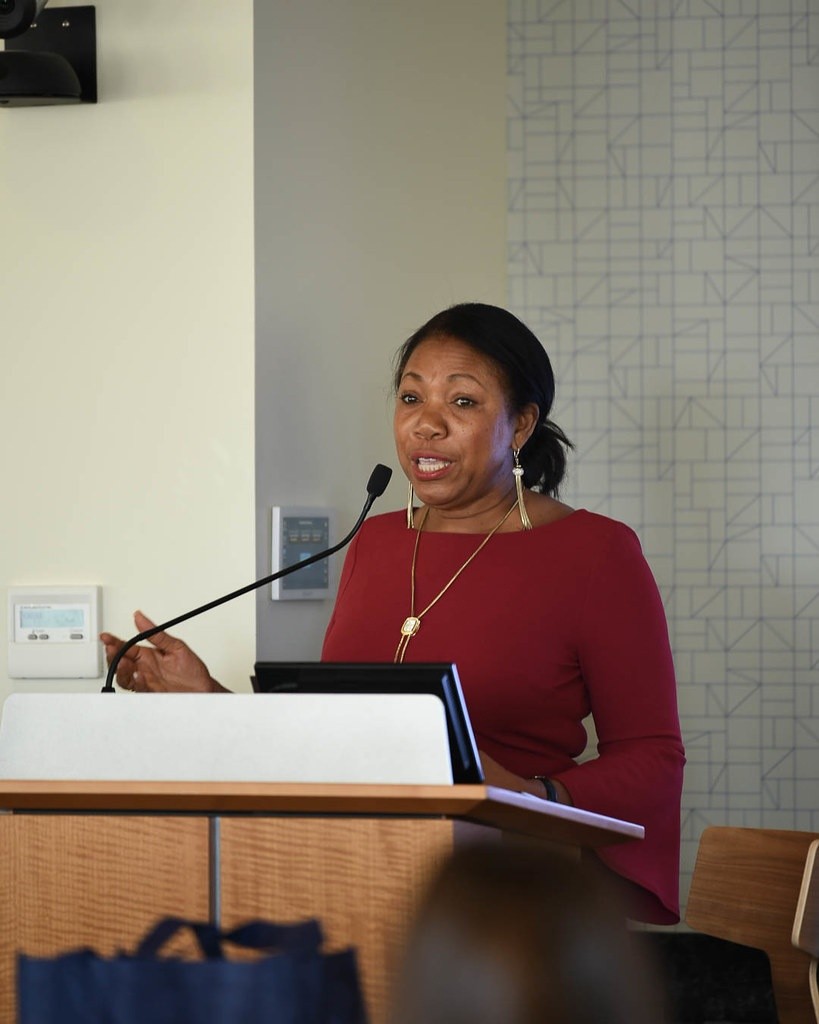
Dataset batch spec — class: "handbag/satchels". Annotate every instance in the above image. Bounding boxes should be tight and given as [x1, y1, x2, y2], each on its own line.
[17, 917, 369, 1024]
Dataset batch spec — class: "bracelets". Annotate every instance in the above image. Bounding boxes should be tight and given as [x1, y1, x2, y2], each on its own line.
[531, 775, 558, 803]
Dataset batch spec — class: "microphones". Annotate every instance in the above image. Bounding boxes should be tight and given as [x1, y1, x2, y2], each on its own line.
[101, 464, 392, 691]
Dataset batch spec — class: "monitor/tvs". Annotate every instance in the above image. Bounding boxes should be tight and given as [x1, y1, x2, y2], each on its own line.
[249, 661, 485, 785]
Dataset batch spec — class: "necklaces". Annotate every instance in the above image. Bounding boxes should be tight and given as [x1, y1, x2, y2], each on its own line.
[394, 486, 526, 664]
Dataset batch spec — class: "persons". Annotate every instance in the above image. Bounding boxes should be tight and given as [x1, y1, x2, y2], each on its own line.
[97, 303, 692, 927]
[384, 834, 684, 1024]
